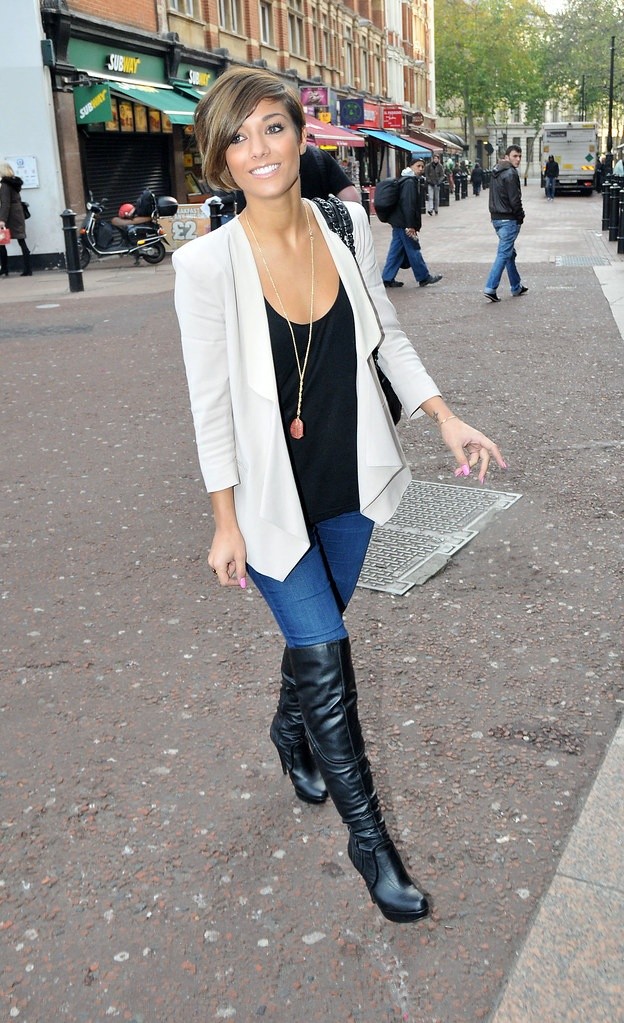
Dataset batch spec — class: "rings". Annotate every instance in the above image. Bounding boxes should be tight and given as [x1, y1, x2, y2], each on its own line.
[212, 568, 218, 574]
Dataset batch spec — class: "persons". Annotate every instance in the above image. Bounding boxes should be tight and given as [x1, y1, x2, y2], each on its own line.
[0, 162, 33, 278]
[543, 154, 559, 201]
[425, 154, 484, 216]
[167, 65, 506, 928]
[594, 154, 607, 193]
[484, 143, 530, 302]
[382, 157, 443, 288]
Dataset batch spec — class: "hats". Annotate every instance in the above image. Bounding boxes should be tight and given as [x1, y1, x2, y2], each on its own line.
[410, 158, 424, 165]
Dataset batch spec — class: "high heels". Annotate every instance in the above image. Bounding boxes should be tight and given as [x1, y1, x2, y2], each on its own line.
[20, 268, 32, 277]
[0, 266, 8, 276]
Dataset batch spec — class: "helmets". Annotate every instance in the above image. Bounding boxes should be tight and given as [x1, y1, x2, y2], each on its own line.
[119, 204, 136, 218]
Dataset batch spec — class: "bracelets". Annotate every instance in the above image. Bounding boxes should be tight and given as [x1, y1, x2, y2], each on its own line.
[440, 415, 457, 425]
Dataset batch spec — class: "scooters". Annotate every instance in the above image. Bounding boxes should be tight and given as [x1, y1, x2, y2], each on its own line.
[79, 188, 180, 266]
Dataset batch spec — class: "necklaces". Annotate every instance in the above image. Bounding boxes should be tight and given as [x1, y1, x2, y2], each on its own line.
[245, 196, 315, 440]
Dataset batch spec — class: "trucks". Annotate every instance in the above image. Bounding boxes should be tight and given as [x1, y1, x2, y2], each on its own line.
[540, 123, 599, 196]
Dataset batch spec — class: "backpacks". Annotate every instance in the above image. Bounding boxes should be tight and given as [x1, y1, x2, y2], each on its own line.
[373, 176, 415, 222]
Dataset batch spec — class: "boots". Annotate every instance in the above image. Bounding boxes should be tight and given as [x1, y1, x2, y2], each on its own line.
[269, 640, 329, 804]
[290, 636, 428, 922]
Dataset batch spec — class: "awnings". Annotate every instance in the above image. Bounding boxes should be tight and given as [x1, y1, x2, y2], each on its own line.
[95, 79, 464, 160]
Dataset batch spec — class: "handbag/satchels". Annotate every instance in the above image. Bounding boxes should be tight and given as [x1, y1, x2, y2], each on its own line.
[21, 201, 30, 218]
[0, 226, 10, 244]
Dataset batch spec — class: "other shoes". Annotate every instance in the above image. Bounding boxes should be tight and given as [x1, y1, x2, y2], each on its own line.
[520, 286, 528, 293]
[428, 211, 432, 215]
[485, 292, 502, 302]
[383, 279, 404, 288]
[435, 211, 438, 214]
[420, 274, 443, 287]
